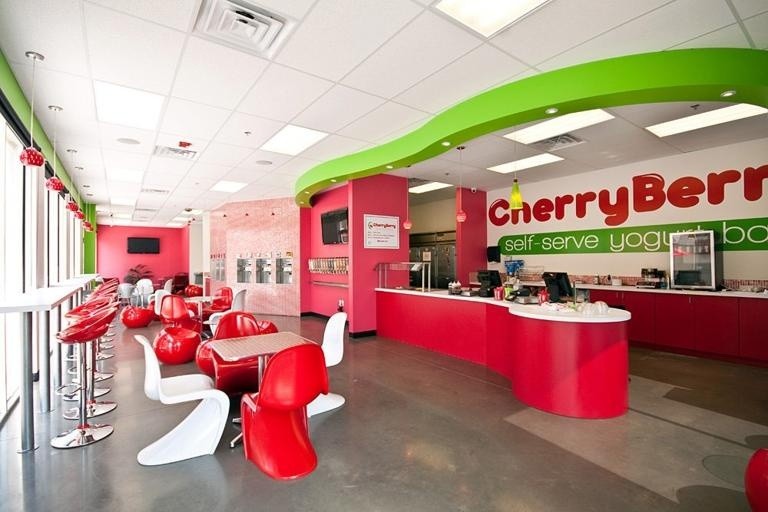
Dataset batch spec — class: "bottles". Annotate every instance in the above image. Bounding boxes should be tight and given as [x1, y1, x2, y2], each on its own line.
[503, 278, 521, 299]
[593, 274, 599, 285]
[448, 280, 462, 296]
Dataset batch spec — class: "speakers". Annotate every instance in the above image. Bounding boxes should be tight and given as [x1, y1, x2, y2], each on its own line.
[487, 247, 500, 262]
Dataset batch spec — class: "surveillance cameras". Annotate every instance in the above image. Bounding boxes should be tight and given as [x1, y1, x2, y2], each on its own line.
[470, 188, 477, 193]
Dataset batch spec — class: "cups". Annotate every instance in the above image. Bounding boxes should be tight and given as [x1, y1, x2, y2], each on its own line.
[582, 301, 609, 315]
[537, 293, 548, 306]
[494, 288, 504, 300]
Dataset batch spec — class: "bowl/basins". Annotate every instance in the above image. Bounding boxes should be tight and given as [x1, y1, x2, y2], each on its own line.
[612, 279, 622, 286]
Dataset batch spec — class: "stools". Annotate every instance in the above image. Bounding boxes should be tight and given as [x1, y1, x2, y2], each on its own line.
[49, 275, 117, 448]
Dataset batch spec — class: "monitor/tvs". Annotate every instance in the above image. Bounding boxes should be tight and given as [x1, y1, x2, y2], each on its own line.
[127, 237, 160, 254]
[542, 272, 573, 303]
[321, 208, 348, 244]
[477, 271, 502, 297]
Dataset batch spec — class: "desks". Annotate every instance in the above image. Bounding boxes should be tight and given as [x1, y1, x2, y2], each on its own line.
[2, 275, 98, 454]
[208, 331, 320, 450]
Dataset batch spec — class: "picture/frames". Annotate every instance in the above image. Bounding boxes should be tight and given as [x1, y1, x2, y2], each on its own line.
[363, 213, 400, 249]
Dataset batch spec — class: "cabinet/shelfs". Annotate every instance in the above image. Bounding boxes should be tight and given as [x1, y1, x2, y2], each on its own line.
[589, 289, 655, 349]
[655, 292, 738, 363]
[738, 298, 767, 370]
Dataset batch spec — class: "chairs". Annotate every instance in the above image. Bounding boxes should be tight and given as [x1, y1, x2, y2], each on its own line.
[238, 343, 330, 480]
[305, 310, 350, 418]
[132, 332, 231, 468]
[210, 311, 264, 390]
[118, 272, 279, 375]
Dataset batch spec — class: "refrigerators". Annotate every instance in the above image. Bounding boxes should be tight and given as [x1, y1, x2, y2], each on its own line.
[669, 230, 721, 291]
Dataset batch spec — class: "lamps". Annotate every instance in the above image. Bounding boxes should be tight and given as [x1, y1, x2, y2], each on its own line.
[403, 167, 412, 230]
[455, 146, 467, 223]
[20, 50, 94, 233]
[508, 124, 524, 211]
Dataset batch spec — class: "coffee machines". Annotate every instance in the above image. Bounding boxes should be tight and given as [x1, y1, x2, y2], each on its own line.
[636, 267, 660, 289]
